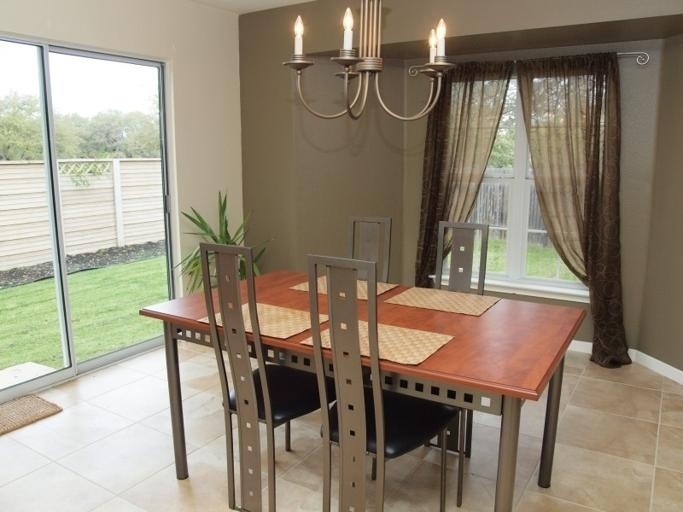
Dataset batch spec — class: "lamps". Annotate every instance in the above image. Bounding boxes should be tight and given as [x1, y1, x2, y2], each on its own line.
[283, 1, 457, 121]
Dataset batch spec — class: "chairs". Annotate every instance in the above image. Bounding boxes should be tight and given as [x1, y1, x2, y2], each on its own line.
[424, 220, 489, 459]
[347, 215, 393, 283]
[308, 255, 466, 512]
[199, 242, 337, 512]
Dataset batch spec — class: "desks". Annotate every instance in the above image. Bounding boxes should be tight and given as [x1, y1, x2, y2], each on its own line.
[139, 271, 587, 512]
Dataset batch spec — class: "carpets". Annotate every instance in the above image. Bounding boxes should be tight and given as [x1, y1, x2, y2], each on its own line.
[0, 393, 63, 437]
